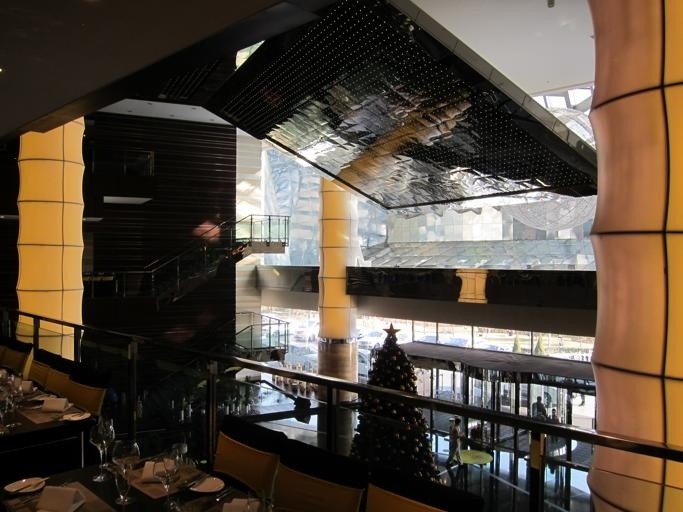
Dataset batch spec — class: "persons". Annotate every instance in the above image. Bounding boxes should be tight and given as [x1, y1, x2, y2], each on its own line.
[442, 414, 465, 466]
[548, 408, 562, 444]
[531, 394, 546, 421]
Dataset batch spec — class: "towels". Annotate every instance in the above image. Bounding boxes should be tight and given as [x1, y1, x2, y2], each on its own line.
[22, 380, 38, 393]
[36, 485, 86, 511]
[42, 398, 74, 412]
[138, 458, 176, 482]
[221, 495, 261, 511]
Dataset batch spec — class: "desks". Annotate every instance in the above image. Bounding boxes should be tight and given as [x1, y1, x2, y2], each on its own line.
[0, 451, 272, 511]
[0, 370, 92, 469]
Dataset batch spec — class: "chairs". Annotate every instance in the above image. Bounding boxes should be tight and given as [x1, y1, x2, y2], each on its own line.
[365, 483, 450, 511]
[269, 465, 366, 511]
[21, 358, 50, 386]
[0, 345, 24, 371]
[212, 431, 281, 499]
[43, 367, 69, 395]
[65, 381, 107, 421]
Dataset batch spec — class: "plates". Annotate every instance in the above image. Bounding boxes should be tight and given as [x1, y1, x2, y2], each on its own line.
[187, 474, 225, 494]
[4, 477, 47, 496]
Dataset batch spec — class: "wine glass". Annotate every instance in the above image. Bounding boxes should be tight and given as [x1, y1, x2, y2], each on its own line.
[151, 451, 183, 511]
[87, 414, 141, 505]
[0, 370, 23, 434]
[242, 498, 274, 512]
